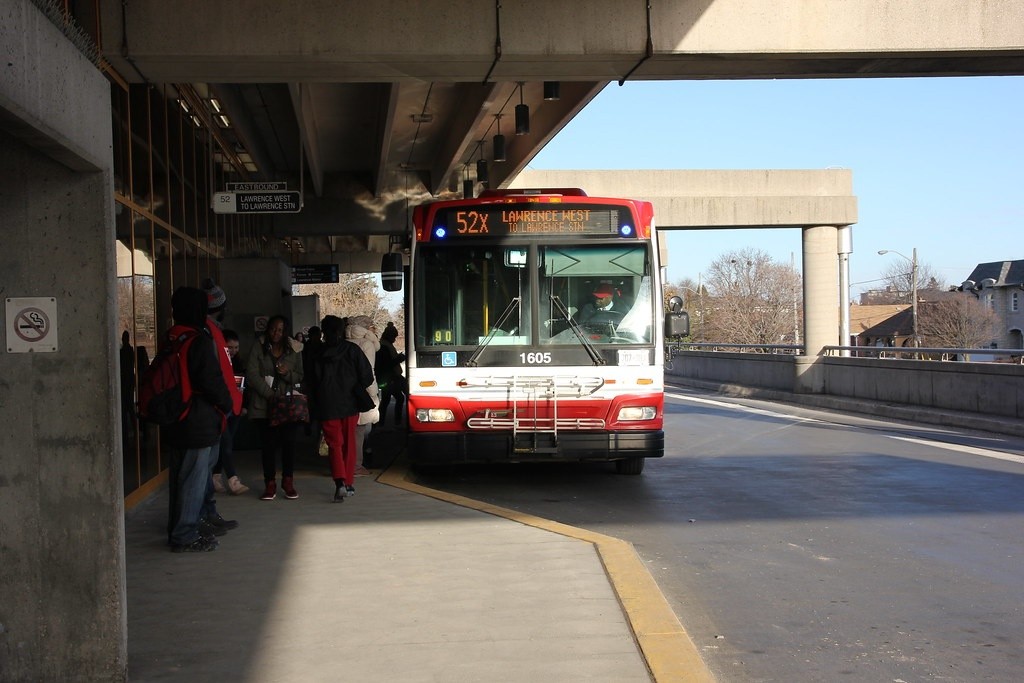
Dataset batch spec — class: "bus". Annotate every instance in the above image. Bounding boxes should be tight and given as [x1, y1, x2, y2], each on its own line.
[379, 186, 692, 477]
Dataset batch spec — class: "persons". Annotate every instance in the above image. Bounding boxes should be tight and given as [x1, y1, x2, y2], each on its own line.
[578, 282, 631, 325]
[295, 315, 374, 503]
[120, 330, 149, 449]
[375, 322, 408, 428]
[243, 315, 304, 500]
[158, 278, 250, 553]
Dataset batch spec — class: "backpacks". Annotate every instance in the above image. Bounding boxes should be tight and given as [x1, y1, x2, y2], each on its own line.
[137, 329, 201, 428]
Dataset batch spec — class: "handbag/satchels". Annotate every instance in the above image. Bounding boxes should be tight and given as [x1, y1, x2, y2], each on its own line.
[267, 369, 310, 428]
[312, 430, 329, 457]
[351, 382, 376, 412]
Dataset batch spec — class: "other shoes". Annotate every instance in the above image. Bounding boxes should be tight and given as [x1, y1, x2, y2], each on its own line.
[393, 423, 405, 432]
[173, 536, 216, 552]
[167, 531, 215, 546]
[334, 484, 346, 503]
[346, 486, 355, 495]
[378, 425, 390, 432]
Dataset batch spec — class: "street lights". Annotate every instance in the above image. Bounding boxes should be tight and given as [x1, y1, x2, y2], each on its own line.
[878, 247, 919, 361]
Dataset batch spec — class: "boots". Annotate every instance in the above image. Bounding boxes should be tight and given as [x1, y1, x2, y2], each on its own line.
[212, 473, 226, 495]
[198, 513, 226, 536]
[206, 500, 239, 531]
[227, 475, 248, 496]
[281, 476, 299, 500]
[261, 479, 276, 500]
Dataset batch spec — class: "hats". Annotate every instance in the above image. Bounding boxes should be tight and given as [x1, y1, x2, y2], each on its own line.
[592, 284, 615, 299]
[385, 322, 399, 336]
[202, 278, 227, 314]
